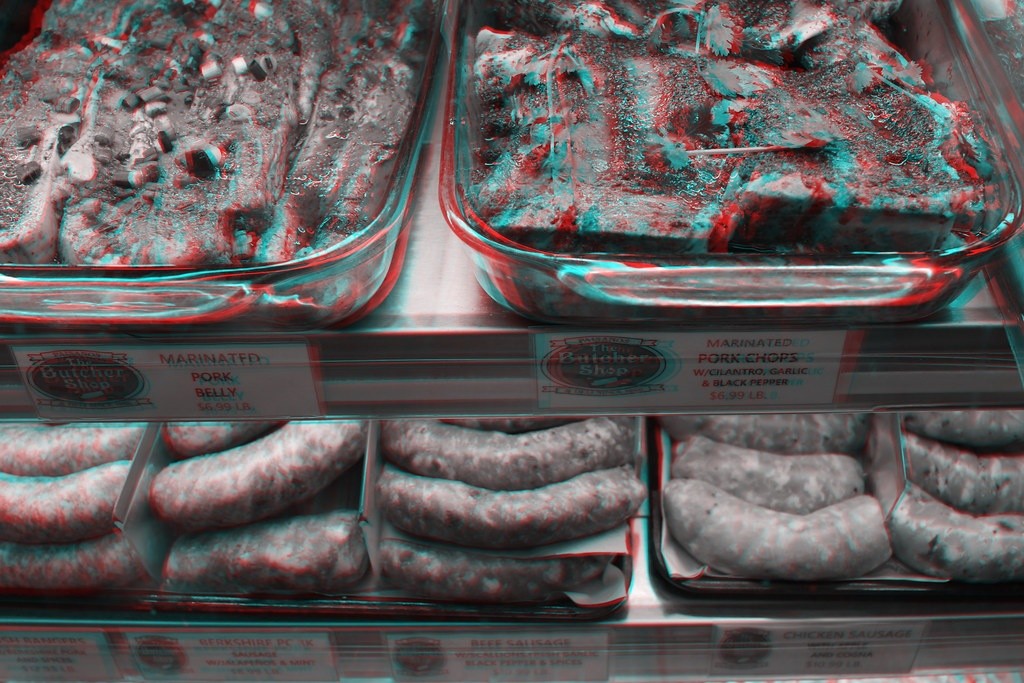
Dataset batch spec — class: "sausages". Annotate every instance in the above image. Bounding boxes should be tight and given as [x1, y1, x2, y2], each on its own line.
[0, 407, 1024, 603]
